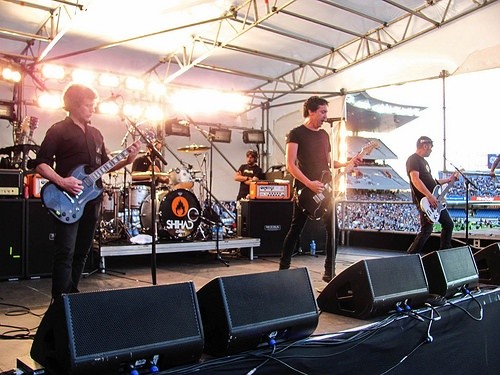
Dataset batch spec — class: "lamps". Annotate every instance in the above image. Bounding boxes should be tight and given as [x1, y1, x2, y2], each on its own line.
[243, 130, 265, 143]
[165, 119, 190, 136]
[210, 129, 232, 143]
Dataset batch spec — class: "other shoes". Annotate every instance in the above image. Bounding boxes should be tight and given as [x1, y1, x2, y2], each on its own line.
[322, 275, 337, 283]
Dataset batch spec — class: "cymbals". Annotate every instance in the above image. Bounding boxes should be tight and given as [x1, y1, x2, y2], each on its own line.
[177, 145, 211, 151]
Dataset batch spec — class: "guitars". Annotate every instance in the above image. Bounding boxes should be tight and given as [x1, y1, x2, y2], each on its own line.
[295, 139, 381, 221]
[40, 129, 157, 225]
[419, 168, 465, 224]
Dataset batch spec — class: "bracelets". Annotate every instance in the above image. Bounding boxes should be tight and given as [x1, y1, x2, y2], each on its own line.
[247, 176, 249, 180]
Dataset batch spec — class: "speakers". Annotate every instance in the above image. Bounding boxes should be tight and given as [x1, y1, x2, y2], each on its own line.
[196, 266, 320, 358]
[30, 280, 205, 375]
[294, 198, 329, 254]
[473, 240, 500, 286]
[238, 199, 295, 258]
[314, 252, 430, 321]
[422, 245, 480, 299]
[0, 199, 59, 279]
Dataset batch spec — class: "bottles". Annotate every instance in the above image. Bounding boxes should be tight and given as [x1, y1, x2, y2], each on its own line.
[212, 225, 217, 240]
[127, 226, 139, 243]
[217, 223, 223, 241]
[310, 240, 316, 256]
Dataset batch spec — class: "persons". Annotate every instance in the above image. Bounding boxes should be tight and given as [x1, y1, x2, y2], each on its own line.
[279, 96, 339, 283]
[132, 144, 162, 172]
[337, 166, 500, 241]
[33, 86, 141, 297]
[406, 137, 455, 255]
[235, 150, 262, 200]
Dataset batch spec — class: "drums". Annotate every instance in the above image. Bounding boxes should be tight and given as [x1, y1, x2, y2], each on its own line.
[167, 167, 194, 190]
[139, 188, 201, 241]
[121, 184, 159, 210]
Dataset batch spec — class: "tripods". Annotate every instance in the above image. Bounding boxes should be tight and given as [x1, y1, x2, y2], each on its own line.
[81, 187, 126, 279]
[198, 215, 231, 266]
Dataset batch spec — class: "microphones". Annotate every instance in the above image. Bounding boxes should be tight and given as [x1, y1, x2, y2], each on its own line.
[323, 117, 345, 122]
[96, 94, 119, 107]
[190, 211, 199, 217]
